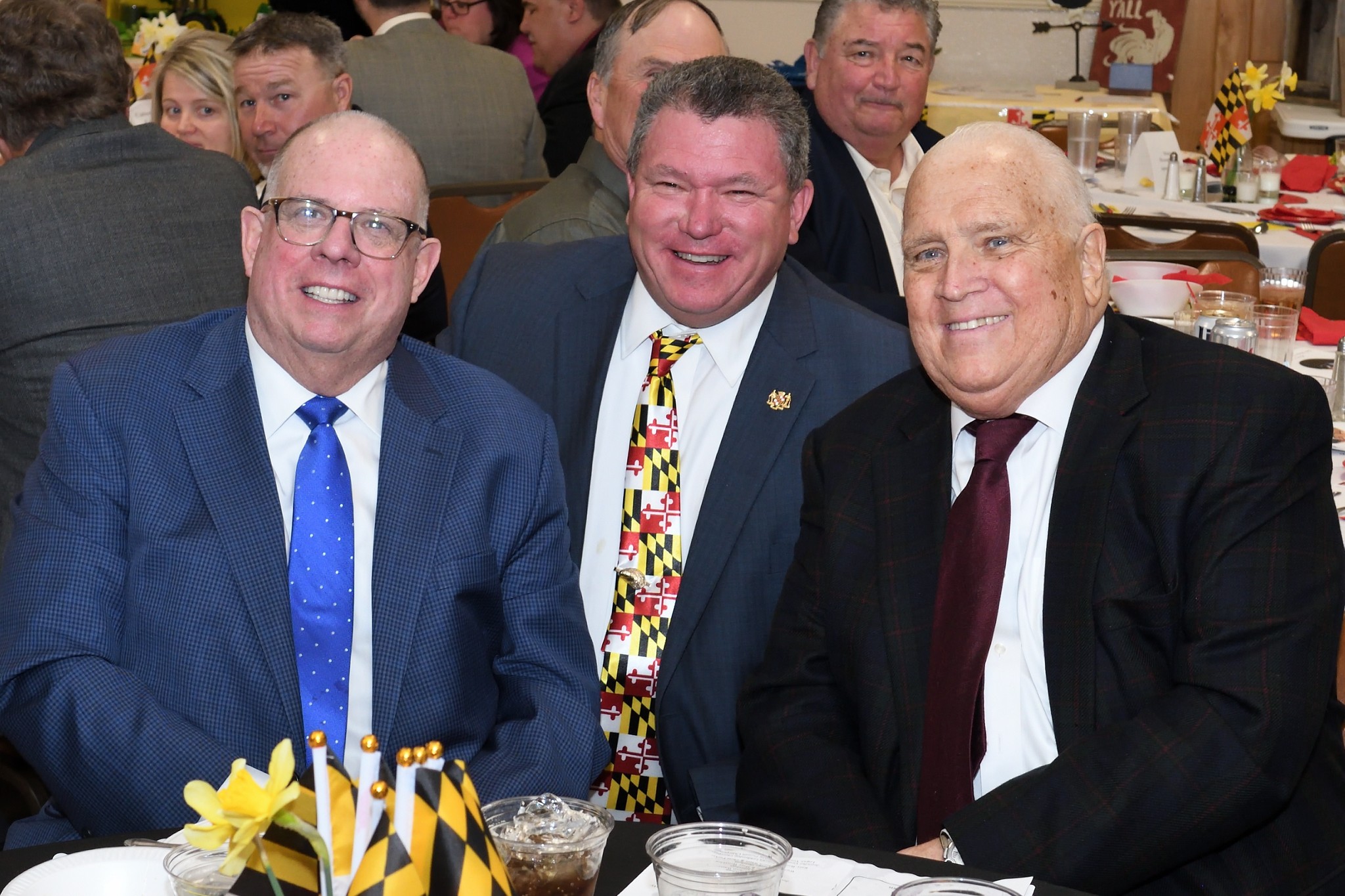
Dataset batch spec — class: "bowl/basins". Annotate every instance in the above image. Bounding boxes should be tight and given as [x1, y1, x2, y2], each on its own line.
[1105, 261, 1204, 319]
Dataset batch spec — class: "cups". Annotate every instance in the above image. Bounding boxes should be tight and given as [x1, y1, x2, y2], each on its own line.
[1245, 304, 1300, 368]
[1067, 112, 1103, 180]
[1310, 375, 1337, 414]
[1258, 165, 1282, 199]
[481, 795, 615, 896]
[1258, 267, 1309, 315]
[890, 877, 1023, 896]
[1114, 134, 1141, 177]
[1189, 289, 1256, 321]
[1335, 138, 1345, 178]
[645, 822, 793, 896]
[1236, 172, 1260, 204]
[163, 835, 265, 896]
[1173, 309, 1201, 336]
[1252, 145, 1288, 174]
[1118, 111, 1152, 144]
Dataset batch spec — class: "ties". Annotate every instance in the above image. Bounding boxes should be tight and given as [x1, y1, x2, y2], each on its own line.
[914, 412, 1040, 845]
[579, 330, 705, 824]
[284, 396, 357, 765]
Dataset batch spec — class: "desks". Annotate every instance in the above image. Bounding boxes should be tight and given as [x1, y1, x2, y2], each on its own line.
[1065, 149, 1345, 269]
[921, 79, 1176, 163]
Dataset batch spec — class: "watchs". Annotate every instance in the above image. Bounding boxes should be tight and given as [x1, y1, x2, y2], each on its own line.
[939, 833, 965, 866]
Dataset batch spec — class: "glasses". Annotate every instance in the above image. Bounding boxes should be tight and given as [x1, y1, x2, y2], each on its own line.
[259, 192, 429, 261]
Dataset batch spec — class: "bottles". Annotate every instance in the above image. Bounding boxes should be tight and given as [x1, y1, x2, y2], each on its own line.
[1191, 157, 1208, 202]
[1161, 151, 1182, 201]
[1328, 335, 1345, 421]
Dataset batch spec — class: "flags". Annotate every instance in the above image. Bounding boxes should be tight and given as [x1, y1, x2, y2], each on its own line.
[229, 732, 511, 896]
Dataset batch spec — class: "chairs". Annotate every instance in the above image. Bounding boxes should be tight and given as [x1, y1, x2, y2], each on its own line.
[1103, 246, 1268, 300]
[424, 172, 554, 305]
[1089, 211, 1262, 260]
[1303, 228, 1345, 319]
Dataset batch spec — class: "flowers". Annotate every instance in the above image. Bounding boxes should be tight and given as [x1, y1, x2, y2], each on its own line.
[133, 9, 188, 57]
[1237, 59, 1300, 119]
[167, 736, 334, 896]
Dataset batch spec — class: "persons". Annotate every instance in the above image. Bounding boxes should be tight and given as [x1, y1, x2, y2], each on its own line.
[432, 56, 916, 826]
[225, 14, 448, 345]
[486, 0, 730, 246]
[338, 0, 621, 208]
[150, 30, 257, 182]
[775, 0, 945, 315]
[0, 112, 610, 846]
[737, 122, 1344, 896]
[0, 0, 259, 548]
